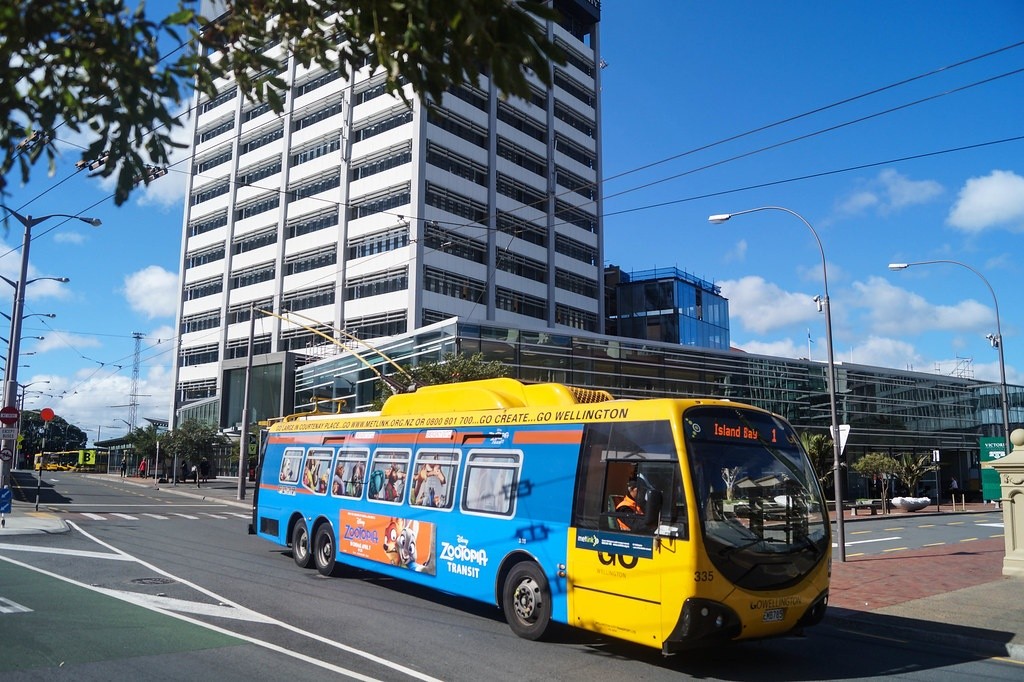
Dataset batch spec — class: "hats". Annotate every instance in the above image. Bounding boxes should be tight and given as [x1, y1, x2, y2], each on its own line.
[628, 477, 651, 491]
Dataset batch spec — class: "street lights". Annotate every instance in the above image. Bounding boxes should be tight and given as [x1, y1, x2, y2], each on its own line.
[887, 260, 1011, 458]
[0, 203, 103, 489]
[707, 205, 846, 563]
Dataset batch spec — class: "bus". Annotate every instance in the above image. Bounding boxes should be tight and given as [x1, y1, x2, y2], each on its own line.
[246, 299, 833, 660]
[33, 448, 109, 473]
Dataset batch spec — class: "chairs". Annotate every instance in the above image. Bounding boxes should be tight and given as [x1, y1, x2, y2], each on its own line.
[607, 494, 629, 531]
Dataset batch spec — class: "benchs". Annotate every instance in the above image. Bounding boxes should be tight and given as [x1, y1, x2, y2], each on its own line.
[848, 504, 881, 516]
[856, 499, 892, 509]
[811, 500, 848, 511]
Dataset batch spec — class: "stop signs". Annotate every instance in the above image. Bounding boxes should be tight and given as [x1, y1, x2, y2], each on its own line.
[0, 406, 20, 425]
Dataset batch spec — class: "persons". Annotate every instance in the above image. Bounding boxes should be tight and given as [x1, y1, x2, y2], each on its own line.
[616, 474, 662, 532]
[180, 460, 187, 483]
[948, 476, 958, 503]
[120, 459, 126, 478]
[200, 457, 210, 483]
[192, 464, 197, 483]
[139, 458, 146, 479]
